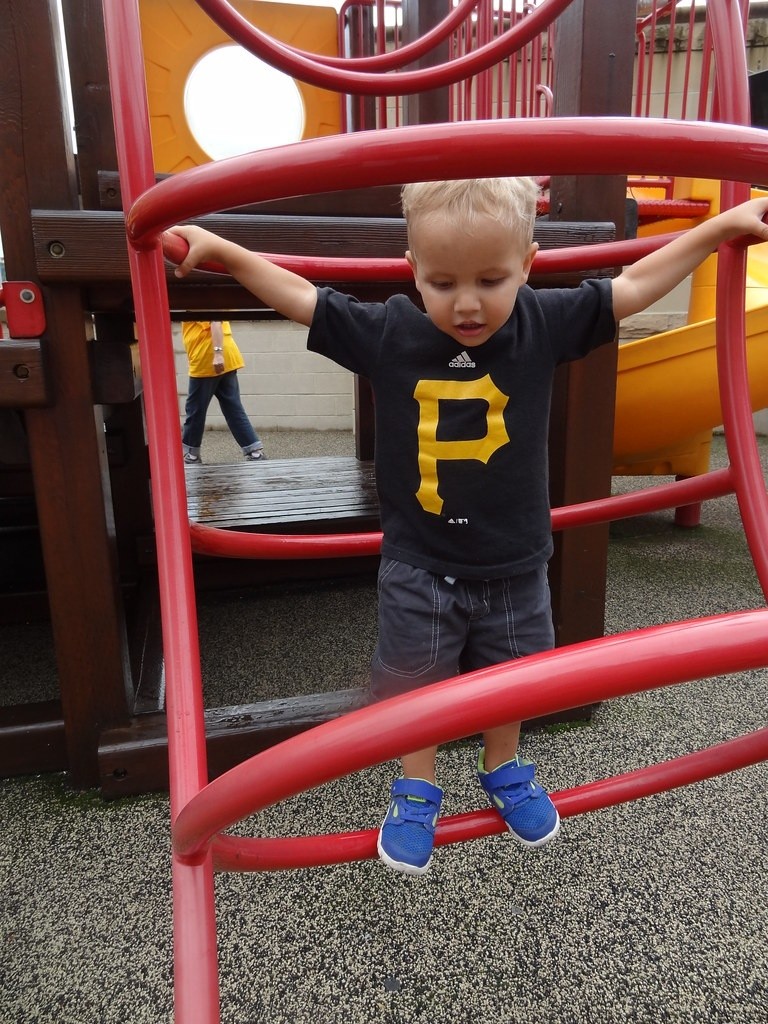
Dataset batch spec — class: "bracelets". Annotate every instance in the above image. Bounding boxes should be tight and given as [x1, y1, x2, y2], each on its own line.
[214, 347, 222, 351]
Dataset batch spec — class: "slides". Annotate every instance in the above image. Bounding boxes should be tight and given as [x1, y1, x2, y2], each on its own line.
[614, 176, 768, 478]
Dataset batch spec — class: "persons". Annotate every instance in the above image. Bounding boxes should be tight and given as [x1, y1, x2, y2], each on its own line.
[182, 321, 267, 464]
[167, 177, 768, 875]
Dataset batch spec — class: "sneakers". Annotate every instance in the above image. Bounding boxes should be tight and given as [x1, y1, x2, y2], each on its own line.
[376, 772, 445, 877]
[476, 744, 560, 847]
[183, 452, 203, 465]
[246, 451, 268, 461]
